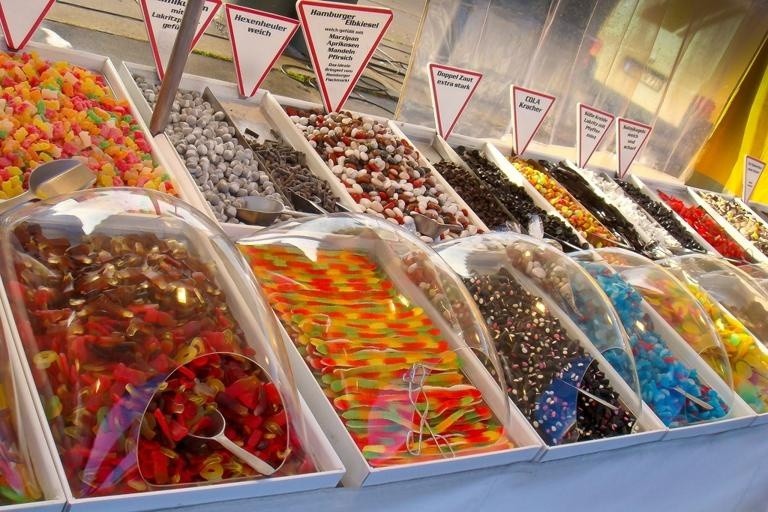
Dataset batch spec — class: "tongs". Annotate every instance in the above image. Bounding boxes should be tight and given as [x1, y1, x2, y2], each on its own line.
[400, 364, 453, 460]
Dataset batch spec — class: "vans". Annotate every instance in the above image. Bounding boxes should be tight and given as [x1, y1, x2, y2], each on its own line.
[578, 0, 752, 146]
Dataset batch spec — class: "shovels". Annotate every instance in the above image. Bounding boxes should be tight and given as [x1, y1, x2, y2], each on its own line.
[414, 214, 461, 239]
[186, 408, 276, 476]
[234, 196, 318, 226]
[0, 160, 97, 216]
[288, 188, 353, 214]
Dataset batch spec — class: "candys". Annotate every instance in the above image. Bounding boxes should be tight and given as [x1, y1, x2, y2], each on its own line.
[0, 382, 43, 507]
[553, 259, 734, 429]
[403, 253, 642, 448]
[655, 190, 750, 258]
[459, 144, 590, 248]
[0, 220, 316, 497]
[630, 276, 768, 415]
[430, 154, 512, 233]
[283, 106, 484, 245]
[0, 47, 181, 202]
[510, 156, 624, 248]
[238, 244, 518, 470]
[616, 179, 707, 250]
[132, 72, 296, 225]
[704, 192, 768, 257]
[538, 159, 645, 249]
[580, 168, 681, 248]
